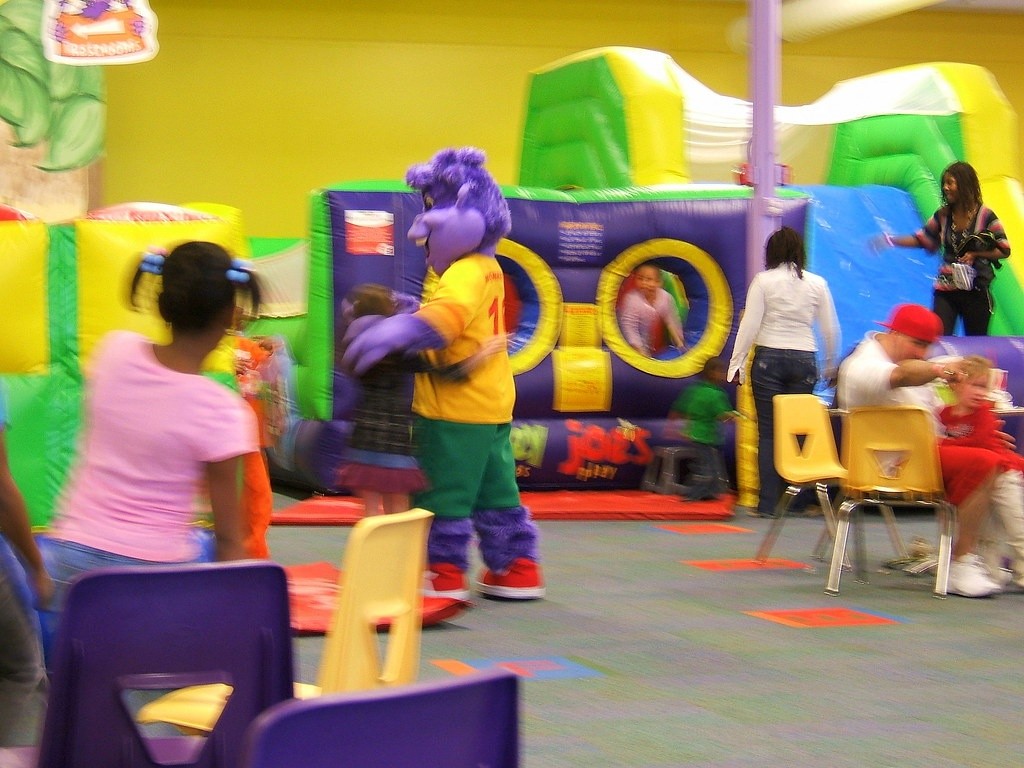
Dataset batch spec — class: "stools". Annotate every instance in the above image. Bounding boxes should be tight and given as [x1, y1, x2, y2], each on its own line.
[644, 445, 728, 496]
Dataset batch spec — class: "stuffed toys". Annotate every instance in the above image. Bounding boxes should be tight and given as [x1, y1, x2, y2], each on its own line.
[340, 146, 544, 600]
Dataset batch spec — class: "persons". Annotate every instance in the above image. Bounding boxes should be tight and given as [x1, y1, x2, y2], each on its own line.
[871, 162, 1011, 336]
[40, 241, 261, 609]
[838, 303, 1016, 598]
[621, 265, 685, 358]
[237, 333, 272, 559]
[0, 382, 55, 747]
[663, 357, 737, 499]
[936, 348, 1024, 587]
[343, 285, 515, 518]
[727, 227, 842, 519]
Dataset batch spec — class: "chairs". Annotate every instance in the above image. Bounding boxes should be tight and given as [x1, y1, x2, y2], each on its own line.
[1, 559, 294, 768]
[237, 672, 518, 768]
[749, 394, 911, 573]
[824, 407, 957, 601]
[134, 508, 434, 739]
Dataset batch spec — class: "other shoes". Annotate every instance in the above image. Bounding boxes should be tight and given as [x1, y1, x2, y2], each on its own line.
[744, 506, 778, 519]
[951, 263, 975, 291]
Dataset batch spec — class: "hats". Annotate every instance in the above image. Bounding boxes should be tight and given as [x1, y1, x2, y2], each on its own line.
[873, 305, 939, 344]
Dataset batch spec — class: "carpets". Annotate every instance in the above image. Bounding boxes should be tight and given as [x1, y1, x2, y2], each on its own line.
[266, 494, 735, 526]
[275, 559, 474, 635]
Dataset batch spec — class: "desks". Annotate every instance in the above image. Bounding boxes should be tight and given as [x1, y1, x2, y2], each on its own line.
[989, 404, 1024, 418]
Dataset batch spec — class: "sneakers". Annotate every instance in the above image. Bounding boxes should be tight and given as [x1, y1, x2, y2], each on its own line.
[947, 553, 1001, 597]
